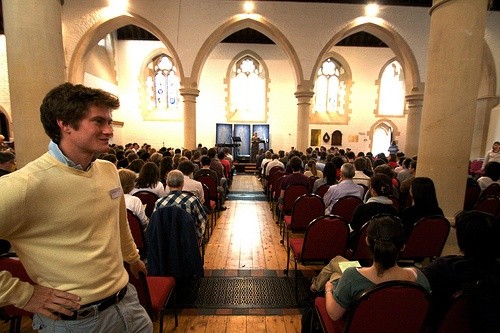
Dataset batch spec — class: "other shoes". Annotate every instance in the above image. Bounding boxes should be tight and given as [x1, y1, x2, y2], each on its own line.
[220, 206, 227, 210]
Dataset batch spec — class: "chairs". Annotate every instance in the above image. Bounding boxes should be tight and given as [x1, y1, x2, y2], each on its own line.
[260, 162, 500, 333]
[126, 162, 229, 333]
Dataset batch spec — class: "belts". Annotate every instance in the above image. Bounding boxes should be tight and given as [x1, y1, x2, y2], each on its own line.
[54, 286, 129, 320]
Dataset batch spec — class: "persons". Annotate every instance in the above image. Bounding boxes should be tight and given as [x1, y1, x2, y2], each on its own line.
[117, 169, 150, 229]
[400, 176, 443, 253]
[463, 140, 500, 211]
[256, 146, 417, 202]
[389, 141, 400, 154]
[349, 174, 403, 253]
[0, 134, 16, 254]
[251, 133, 261, 163]
[99, 143, 234, 211]
[310, 214, 431, 322]
[151, 168, 208, 247]
[0, 83, 154, 333]
[324, 163, 365, 217]
[432, 210, 500, 333]
